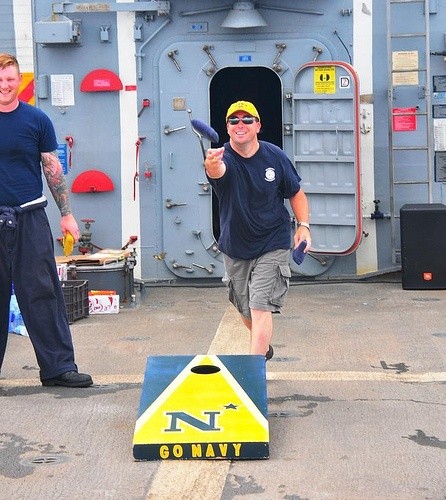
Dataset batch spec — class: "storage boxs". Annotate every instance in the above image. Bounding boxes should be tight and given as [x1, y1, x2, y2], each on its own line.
[60, 279, 89, 324]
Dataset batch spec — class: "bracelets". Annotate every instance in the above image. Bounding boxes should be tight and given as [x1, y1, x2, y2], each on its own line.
[297, 222, 310, 232]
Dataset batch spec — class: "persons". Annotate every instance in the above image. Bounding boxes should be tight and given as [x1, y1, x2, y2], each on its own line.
[205, 100, 312, 359]
[0, 52, 94, 389]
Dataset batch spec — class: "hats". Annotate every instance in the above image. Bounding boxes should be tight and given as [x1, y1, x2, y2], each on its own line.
[225, 100, 260, 123]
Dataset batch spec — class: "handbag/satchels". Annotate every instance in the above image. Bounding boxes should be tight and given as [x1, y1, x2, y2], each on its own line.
[7, 293, 27, 335]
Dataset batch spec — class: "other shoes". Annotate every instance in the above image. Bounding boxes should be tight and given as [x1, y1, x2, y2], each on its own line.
[42, 370, 93, 386]
[265, 345, 273, 359]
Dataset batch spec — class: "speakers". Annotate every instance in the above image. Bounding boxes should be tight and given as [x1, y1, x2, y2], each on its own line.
[399, 203, 446, 290]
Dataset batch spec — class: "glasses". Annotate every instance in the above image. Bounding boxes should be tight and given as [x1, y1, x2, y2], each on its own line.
[226, 115, 255, 125]
